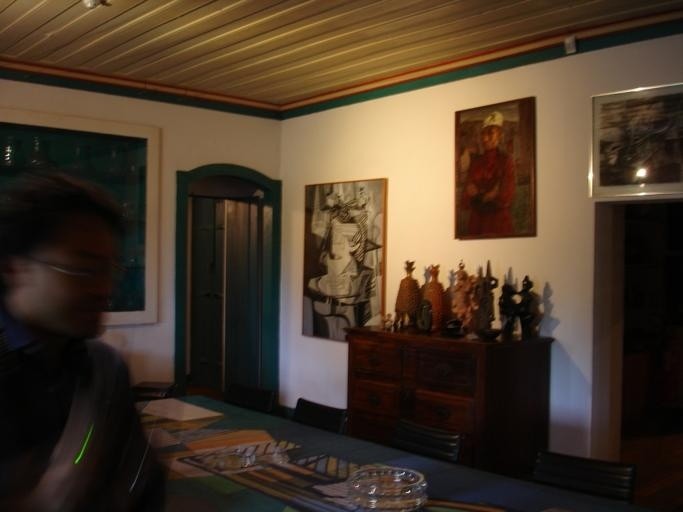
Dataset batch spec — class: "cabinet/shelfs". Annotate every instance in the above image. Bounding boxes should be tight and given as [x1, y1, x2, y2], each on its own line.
[342, 321, 554, 458]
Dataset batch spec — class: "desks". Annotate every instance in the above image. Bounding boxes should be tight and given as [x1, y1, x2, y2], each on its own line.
[134, 390, 636, 512]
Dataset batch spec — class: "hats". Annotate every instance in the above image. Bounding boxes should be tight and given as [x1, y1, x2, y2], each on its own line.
[483, 111, 504, 128]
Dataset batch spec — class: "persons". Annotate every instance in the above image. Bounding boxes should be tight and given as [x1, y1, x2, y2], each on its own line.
[2, 165, 165, 510]
[460, 112, 516, 236]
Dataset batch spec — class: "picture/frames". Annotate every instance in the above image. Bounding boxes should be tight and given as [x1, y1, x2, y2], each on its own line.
[301, 178, 388, 344]
[0, 108, 161, 327]
[588, 82, 683, 198]
[453, 95, 536, 237]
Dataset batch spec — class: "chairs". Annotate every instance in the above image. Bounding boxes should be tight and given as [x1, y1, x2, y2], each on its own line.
[531, 448, 636, 505]
[293, 397, 347, 436]
[395, 418, 464, 466]
[222, 382, 272, 413]
